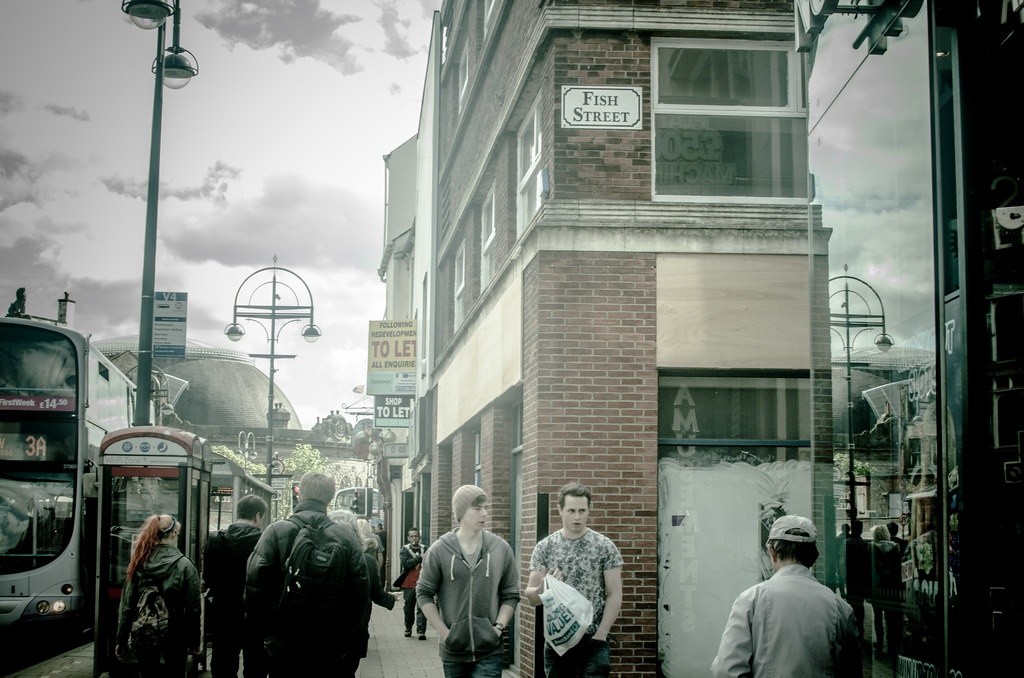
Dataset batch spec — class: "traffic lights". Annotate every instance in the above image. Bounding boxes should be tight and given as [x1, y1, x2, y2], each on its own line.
[350, 487, 367, 517]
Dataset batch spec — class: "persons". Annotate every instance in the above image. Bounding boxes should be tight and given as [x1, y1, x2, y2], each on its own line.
[525, 482, 623, 678]
[710, 515, 859, 678]
[330, 504, 402, 623]
[836, 523, 913, 668]
[414, 484, 521, 678]
[115, 514, 201, 678]
[245, 470, 369, 678]
[201, 495, 268, 678]
[393, 527, 429, 640]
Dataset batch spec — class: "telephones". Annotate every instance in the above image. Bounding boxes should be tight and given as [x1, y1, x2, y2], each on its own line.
[110, 527, 140, 584]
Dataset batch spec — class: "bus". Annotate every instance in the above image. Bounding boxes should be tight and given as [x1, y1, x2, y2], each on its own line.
[0, 288, 155, 624]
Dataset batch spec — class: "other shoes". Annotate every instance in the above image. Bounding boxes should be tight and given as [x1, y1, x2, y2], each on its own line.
[404, 626, 412, 636]
[418, 633, 426, 640]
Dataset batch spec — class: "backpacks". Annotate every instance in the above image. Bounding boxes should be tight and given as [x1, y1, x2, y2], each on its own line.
[128, 553, 186, 654]
[263, 517, 346, 642]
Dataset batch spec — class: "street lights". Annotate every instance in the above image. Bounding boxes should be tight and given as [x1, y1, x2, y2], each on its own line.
[827, 264, 894, 531]
[224, 255, 322, 486]
[122, 0, 200, 430]
[235, 431, 257, 461]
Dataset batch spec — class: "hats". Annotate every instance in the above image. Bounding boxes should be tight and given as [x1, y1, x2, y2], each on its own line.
[766, 515, 816, 543]
[452, 485, 487, 522]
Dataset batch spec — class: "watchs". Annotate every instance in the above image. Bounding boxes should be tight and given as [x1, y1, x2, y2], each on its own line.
[492, 623, 504, 632]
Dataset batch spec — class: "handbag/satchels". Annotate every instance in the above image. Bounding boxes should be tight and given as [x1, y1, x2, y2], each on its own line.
[539, 573, 594, 657]
